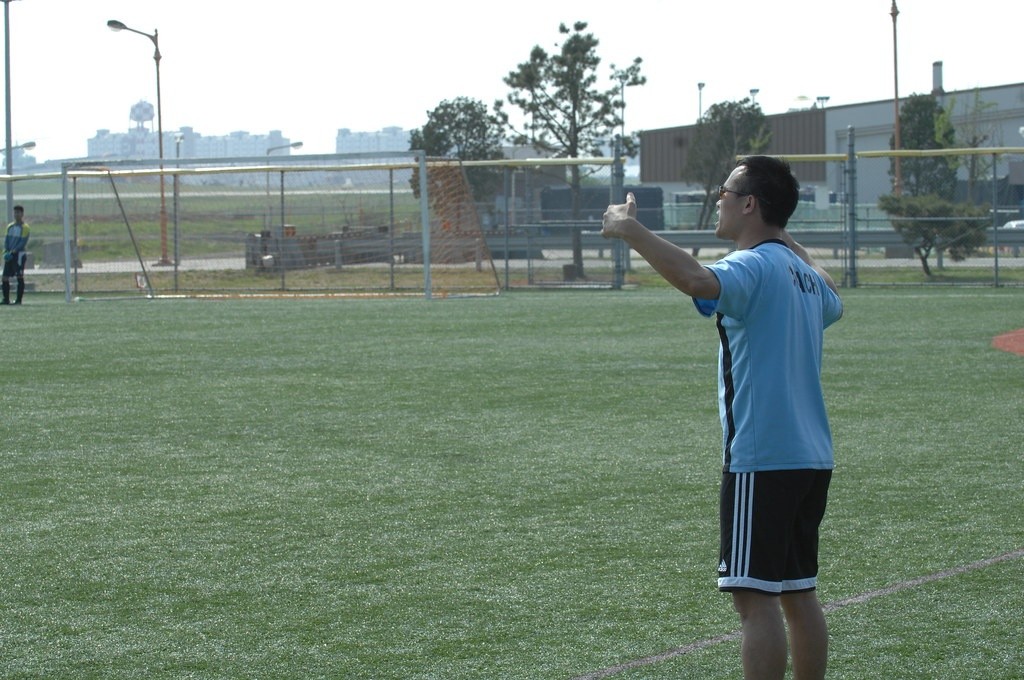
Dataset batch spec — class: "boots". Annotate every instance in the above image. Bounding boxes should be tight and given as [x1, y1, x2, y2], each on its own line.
[10, 283, 25, 305]
[0, 283, 9, 305]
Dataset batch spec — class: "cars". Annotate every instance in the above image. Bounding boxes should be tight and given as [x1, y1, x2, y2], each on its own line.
[1003, 220, 1024, 228]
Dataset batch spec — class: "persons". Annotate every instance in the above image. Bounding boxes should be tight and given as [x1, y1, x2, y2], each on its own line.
[0, 205, 30, 306]
[600, 155, 843, 680]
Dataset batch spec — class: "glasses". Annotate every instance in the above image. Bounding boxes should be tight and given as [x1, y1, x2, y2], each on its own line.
[718, 185, 750, 199]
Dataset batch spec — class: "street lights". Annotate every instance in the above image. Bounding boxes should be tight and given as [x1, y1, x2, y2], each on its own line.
[620, 75, 625, 146]
[106, 19, 168, 265]
[698, 84, 705, 124]
[263, 140, 301, 230]
[818, 97, 830, 107]
[175, 135, 183, 224]
[751, 88, 760, 110]
